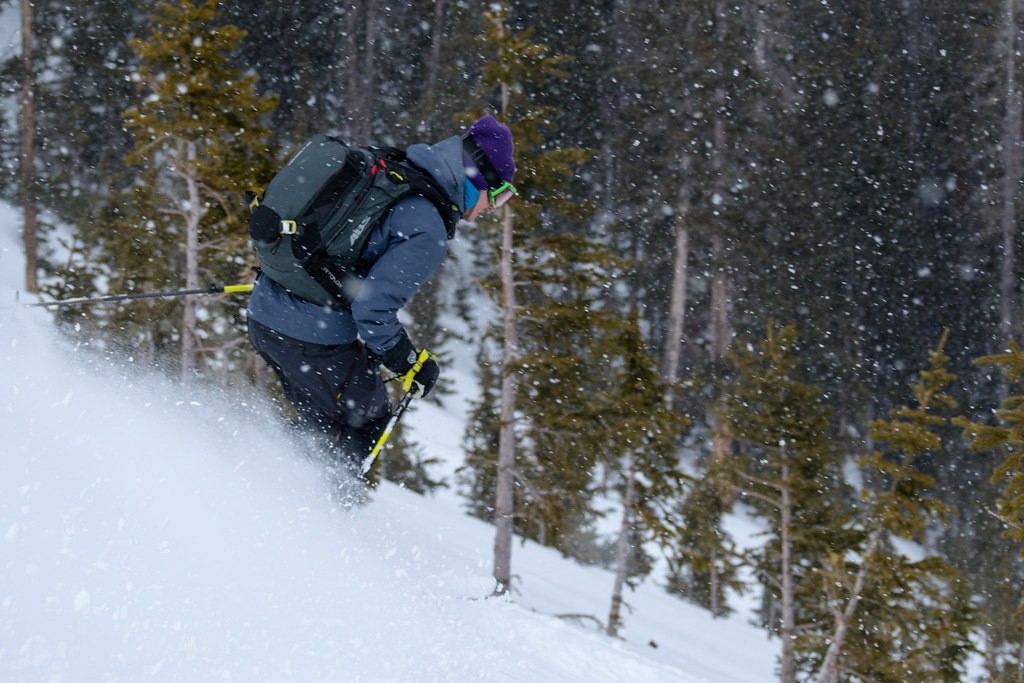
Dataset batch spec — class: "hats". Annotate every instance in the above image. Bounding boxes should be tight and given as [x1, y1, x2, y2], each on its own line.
[464, 115, 516, 192]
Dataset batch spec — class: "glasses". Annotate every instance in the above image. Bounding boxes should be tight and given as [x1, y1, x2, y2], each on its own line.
[488, 182, 515, 210]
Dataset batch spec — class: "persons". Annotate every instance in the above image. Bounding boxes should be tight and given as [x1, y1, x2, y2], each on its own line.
[245, 111, 516, 514]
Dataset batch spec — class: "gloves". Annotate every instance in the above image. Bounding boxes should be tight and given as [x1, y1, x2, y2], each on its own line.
[365, 327, 439, 400]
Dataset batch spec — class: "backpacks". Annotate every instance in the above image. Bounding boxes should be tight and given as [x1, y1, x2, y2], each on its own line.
[247, 132, 457, 311]
